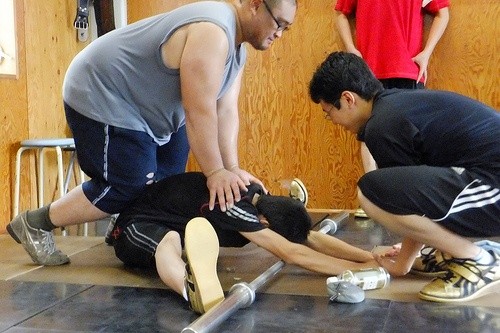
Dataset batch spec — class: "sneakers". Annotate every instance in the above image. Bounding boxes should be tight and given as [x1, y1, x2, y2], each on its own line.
[289, 178, 308, 209]
[410, 247, 454, 275]
[6, 209, 70, 266]
[417, 250, 500, 303]
[184, 217, 224, 314]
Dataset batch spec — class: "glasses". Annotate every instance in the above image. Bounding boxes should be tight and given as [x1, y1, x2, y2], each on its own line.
[325, 100, 339, 120]
[263, 0, 288, 30]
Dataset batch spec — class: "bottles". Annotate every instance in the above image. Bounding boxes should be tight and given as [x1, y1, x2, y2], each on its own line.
[341, 267, 391, 291]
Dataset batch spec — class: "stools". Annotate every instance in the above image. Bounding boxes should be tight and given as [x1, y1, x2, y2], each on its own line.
[13, 137, 88, 236]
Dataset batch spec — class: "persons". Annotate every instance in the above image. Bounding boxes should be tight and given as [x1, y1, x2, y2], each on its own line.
[6, 0, 298, 267]
[334, 0, 451, 219]
[112, 171, 400, 316]
[308, 50, 500, 303]
